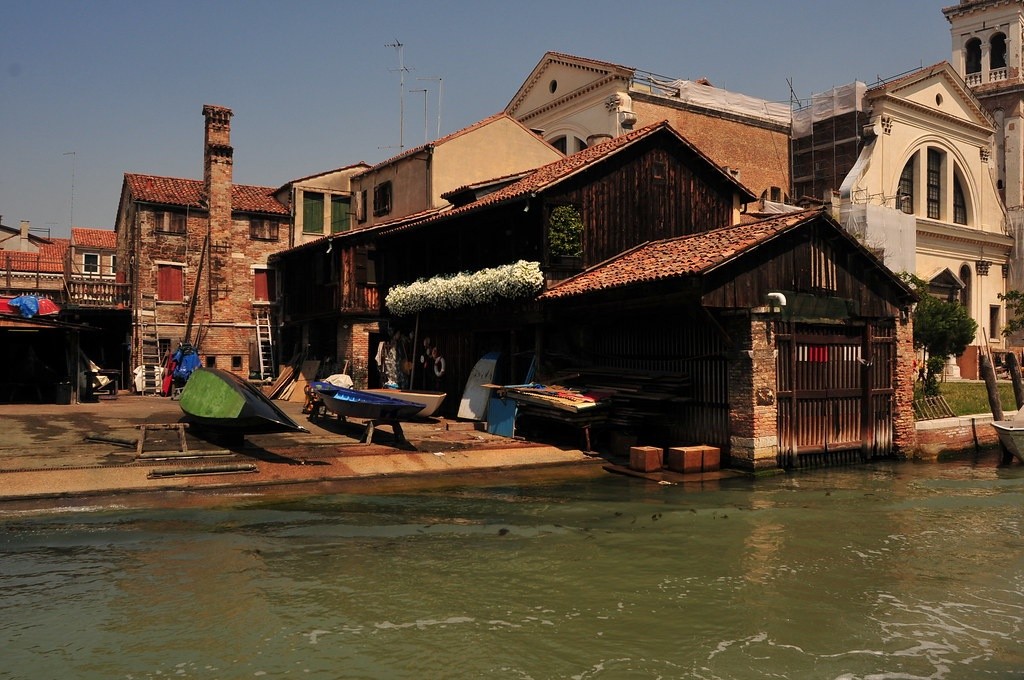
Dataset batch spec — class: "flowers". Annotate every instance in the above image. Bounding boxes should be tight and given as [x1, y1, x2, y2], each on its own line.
[384, 258, 545, 318]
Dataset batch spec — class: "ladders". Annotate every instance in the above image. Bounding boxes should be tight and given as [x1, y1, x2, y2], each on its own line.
[141, 293, 163, 397]
[256, 313, 276, 380]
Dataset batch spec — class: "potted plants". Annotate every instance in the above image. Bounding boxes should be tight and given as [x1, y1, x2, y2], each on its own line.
[546, 204, 587, 268]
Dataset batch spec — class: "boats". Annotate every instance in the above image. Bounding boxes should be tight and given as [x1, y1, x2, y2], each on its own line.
[310, 379, 426, 422]
[180, 367, 312, 435]
[357, 388, 448, 419]
